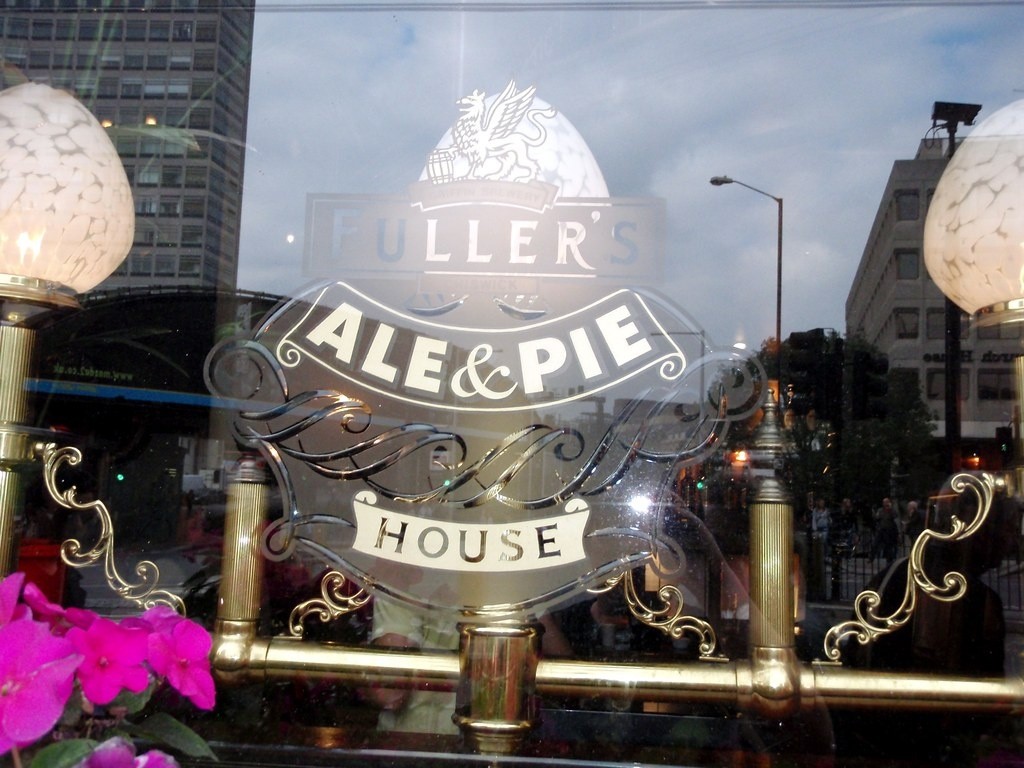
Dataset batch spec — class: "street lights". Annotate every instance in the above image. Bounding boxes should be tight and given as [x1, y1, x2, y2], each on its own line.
[929, 99, 986, 487]
[650, 329, 708, 517]
[709, 174, 785, 381]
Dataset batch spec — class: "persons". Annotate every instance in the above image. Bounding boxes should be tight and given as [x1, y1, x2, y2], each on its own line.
[356, 447, 573, 735]
[14, 469, 218, 611]
[713, 472, 1015, 768]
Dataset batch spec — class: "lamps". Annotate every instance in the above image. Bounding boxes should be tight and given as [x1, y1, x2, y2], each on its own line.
[0, 78, 135, 326]
[922, 97, 1024, 353]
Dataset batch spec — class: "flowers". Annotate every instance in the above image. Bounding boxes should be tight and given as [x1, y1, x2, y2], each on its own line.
[0, 568, 221, 768]
